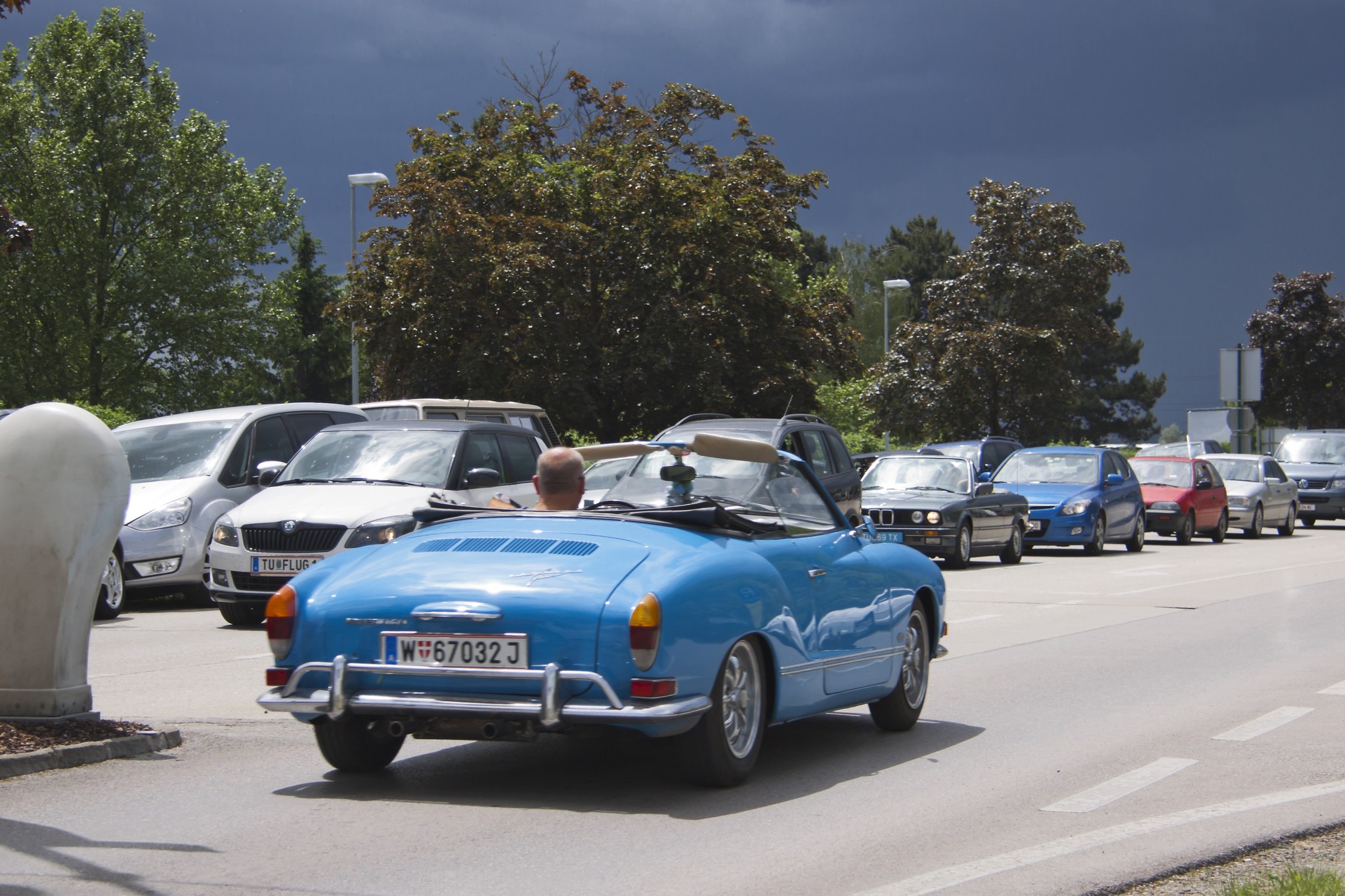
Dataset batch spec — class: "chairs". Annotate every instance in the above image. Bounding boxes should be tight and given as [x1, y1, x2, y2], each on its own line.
[462, 443, 484, 469]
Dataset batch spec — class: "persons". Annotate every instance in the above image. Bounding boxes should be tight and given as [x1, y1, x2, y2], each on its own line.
[945, 467, 962, 487]
[487, 445, 585, 511]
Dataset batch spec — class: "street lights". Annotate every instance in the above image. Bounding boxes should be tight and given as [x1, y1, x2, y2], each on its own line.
[347, 171, 390, 405]
[882, 278, 911, 451]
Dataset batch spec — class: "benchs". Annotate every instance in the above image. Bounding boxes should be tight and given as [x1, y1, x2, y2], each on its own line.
[790, 436, 826, 478]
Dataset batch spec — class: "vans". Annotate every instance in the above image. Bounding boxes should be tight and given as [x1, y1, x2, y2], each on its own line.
[1271, 428, 1345, 529]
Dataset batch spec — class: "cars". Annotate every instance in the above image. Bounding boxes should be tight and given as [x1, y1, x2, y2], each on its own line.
[209, 417, 550, 625]
[1127, 455, 1229, 546]
[89, 399, 377, 621]
[1195, 452, 1301, 540]
[979, 445, 1148, 556]
[344, 398, 1029, 513]
[1135, 439, 1225, 459]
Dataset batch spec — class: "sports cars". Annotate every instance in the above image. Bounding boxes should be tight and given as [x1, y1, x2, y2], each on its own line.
[857, 454, 1031, 569]
[252, 440, 950, 791]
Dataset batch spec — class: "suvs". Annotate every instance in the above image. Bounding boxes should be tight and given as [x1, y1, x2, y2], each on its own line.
[599, 411, 865, 534]
